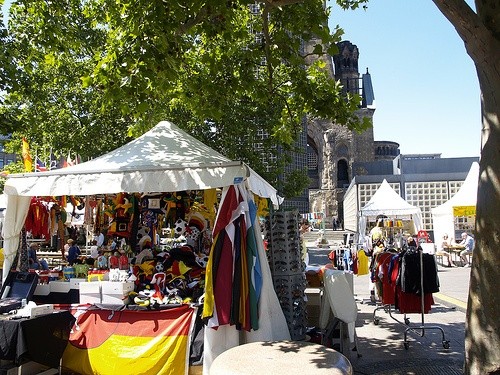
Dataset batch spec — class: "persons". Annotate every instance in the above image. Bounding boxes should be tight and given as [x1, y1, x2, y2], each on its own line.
[28, 229, 106, 270]
[333, 219, 336, 231]
[455, 233, 474, 267]
[438, 233, 458, 268]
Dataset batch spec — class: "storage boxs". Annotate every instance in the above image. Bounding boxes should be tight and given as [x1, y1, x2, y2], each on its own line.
[33, 278, 135, 305]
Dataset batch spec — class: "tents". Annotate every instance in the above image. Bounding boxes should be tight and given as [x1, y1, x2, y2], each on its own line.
[3, 122, 284, 375]
[360, 161, 481, 266]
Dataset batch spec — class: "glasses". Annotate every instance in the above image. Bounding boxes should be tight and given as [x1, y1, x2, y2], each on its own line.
[259, 208, 308, 335]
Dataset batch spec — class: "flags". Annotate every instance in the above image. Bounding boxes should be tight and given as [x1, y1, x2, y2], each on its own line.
[23, 140, 89, 208]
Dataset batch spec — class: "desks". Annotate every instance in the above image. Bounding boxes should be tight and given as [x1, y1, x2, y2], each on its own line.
[0, 309, 76, 375]
[444, 245, 461, 267]
[210, 340, 354, 375]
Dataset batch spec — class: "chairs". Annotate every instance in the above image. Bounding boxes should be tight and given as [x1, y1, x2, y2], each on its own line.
[433, 243, 445, 265]
